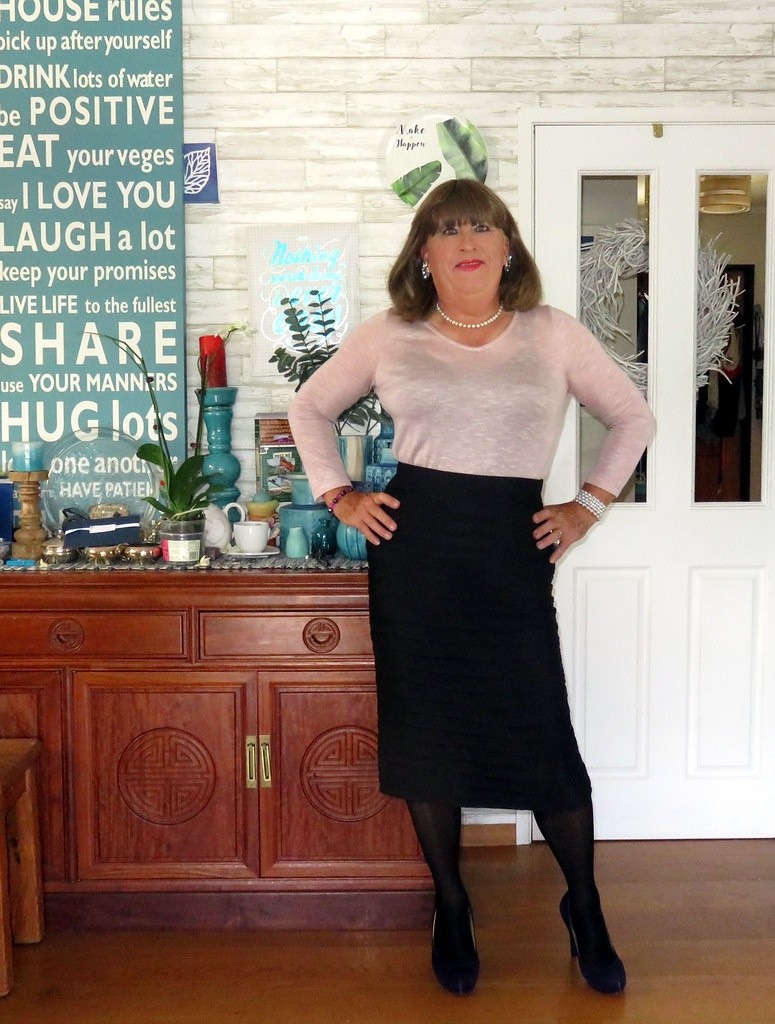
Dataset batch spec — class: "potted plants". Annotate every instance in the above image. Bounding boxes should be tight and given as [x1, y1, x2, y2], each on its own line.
[269, 289, 393, 482]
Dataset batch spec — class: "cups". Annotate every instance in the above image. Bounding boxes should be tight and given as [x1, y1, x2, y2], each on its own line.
[233, 521, 270, 552]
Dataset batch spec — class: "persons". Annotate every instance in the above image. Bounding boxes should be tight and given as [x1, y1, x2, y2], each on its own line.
[287, 179, 657, 996]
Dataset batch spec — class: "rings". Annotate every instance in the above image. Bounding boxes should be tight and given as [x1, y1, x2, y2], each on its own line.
[558, 529, 564, 537]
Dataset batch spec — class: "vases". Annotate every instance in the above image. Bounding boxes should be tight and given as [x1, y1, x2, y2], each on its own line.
[159, 515, 207, 565]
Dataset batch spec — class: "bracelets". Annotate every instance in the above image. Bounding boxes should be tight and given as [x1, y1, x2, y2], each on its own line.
[575, 489, 607, 521]
[328, 487, 354, 512]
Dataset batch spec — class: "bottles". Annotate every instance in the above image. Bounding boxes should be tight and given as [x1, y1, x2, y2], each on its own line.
[312, 520, 336, 557]
[285, 527, 308, 558]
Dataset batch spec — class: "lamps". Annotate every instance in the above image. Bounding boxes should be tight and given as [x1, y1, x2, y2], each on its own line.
[700, 175, 753, 215]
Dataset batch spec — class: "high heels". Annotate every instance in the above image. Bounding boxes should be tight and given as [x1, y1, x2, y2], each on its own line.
[427, 895, 479, 995]
[559, 891, 626, 995]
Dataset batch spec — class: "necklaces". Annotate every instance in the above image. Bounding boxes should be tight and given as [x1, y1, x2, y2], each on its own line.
[437, 301, 503, 328]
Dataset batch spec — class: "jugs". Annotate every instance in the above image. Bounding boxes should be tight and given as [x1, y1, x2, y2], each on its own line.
[202, 503, 245, 549]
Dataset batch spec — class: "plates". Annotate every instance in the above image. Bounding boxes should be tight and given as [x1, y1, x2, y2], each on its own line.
[229, 545, 280, 558]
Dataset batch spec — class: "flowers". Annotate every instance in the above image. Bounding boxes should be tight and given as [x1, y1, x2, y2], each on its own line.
[78, 322, 259, 519]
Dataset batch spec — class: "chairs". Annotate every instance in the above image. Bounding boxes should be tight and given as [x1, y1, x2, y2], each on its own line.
[0, 738, 46, 997]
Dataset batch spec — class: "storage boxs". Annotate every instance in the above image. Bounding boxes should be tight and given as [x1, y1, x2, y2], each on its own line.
[63, 516, 140, 549]
[255, 412, 306, 503]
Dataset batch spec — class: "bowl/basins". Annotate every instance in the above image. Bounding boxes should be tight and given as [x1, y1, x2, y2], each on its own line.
[78, 543, 125, 565]
[125, 542, 162, 565]
[41, 548, 74, 564]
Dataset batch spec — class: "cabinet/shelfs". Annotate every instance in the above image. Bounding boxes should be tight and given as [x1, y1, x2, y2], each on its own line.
[0, 554, 437, 932]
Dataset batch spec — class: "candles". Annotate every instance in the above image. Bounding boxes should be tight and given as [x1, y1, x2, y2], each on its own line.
[199, 334, 228, 388]
